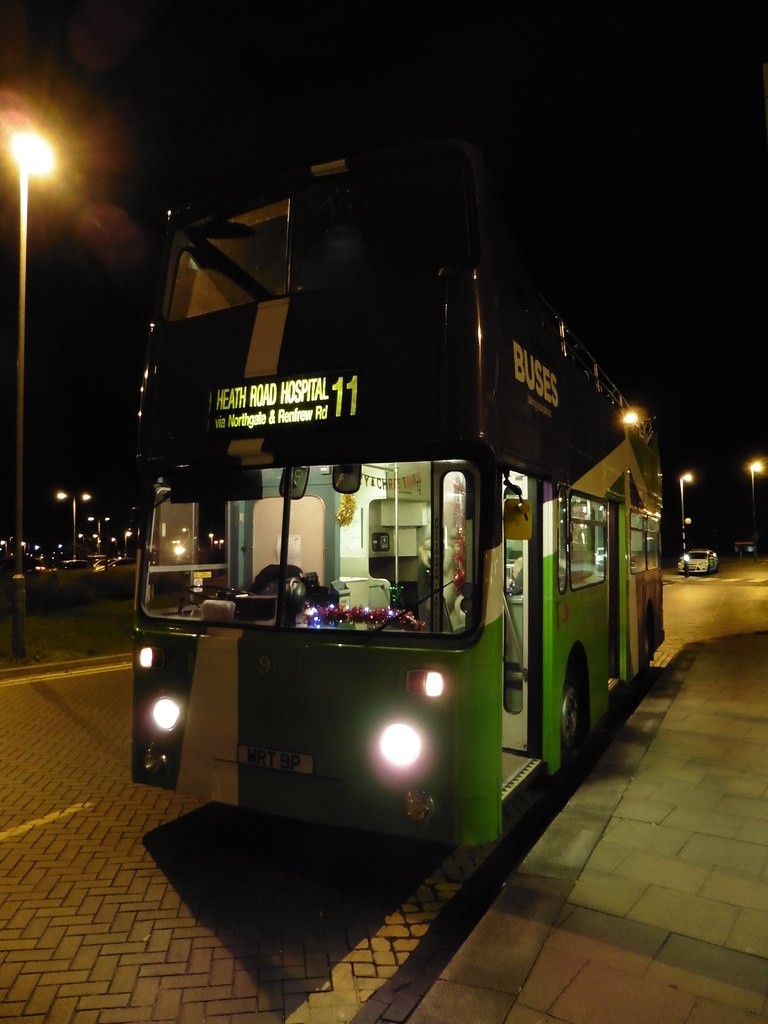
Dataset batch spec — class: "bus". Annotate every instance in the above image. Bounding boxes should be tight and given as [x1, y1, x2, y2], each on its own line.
[127, 131, 664, 849]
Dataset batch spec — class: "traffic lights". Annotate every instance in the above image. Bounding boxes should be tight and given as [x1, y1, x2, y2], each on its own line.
[0, 531, 133, 555]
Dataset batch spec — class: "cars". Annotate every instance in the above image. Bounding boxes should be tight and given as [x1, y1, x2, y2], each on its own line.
[45, 558, 135, 574]
[677, 549, 719, 575]
[1, 555, 45, 575]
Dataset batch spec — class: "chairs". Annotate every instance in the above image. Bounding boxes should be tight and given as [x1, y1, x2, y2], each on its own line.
[256, 565, 303, 595]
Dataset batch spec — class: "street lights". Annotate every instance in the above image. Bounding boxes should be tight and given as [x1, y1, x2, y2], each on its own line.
[2, 107, 77, 659]
[749, 459, 767, 559]
[680, 473, 695, 577]
[55, 488, 92, 559]
[87, 516, 111, 555]
[209, 532, 224, 551]
